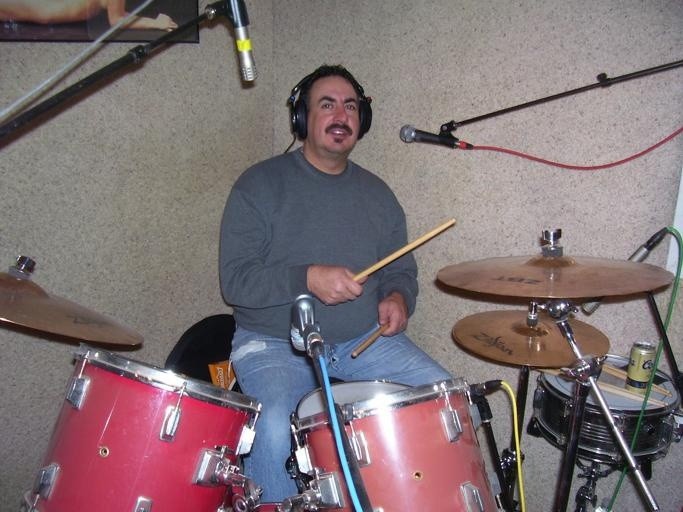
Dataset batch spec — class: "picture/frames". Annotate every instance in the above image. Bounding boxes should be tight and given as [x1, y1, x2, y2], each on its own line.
[1, 0, 200, 43]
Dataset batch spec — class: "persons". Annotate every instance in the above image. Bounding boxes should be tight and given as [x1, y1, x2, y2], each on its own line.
[218, 62, 483, 506]
[0, 1, 176, 34]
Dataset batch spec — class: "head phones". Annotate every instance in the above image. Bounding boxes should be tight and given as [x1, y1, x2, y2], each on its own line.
[288, 65, 374, 140]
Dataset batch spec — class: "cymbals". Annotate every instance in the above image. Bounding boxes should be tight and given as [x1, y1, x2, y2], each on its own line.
[0, 270, 144, 344]
[435, 256, 675, 300]
[451, 309, 609, 369]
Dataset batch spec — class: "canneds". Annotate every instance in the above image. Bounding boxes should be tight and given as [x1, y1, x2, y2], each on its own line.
[623, 341, 656, 397]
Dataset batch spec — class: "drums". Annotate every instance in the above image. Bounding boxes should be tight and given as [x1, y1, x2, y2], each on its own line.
[535, 355, 676, 463]
[23, 340, 262, 511]
[298, 377, 417, 428]
[289, 377, 499, 512]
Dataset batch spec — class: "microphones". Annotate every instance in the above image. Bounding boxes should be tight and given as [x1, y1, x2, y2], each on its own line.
[400, 124, 475, 151]
[470, 378, 503, 400]
[581, 228, 668, 316]
[289, 294, 317, 354]
[225, 0, 258, 84]
